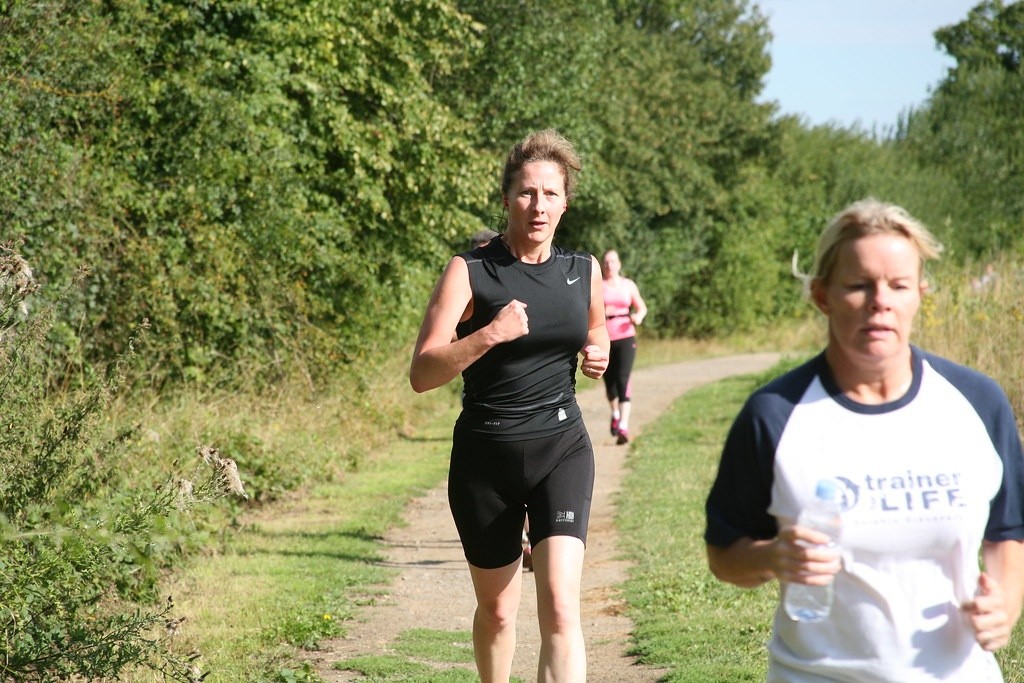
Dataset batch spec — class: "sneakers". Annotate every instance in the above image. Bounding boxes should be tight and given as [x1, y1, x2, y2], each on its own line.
[611, 418, 629, 442]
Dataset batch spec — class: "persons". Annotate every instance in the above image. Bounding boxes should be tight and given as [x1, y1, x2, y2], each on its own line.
[587, 249, 647, 442]
[704, 196, 1023, 682]
[466, 227, 532, 572]
[411, 129, 610, 683]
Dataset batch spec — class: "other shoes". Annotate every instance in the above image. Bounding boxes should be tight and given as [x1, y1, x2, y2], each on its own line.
[523, 545, 534, 572]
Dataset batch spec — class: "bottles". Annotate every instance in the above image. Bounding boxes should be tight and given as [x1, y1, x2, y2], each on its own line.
[785, 480, 842, 624]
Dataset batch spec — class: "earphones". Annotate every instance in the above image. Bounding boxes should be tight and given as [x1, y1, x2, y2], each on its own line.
[504, 200, 508, 207]
